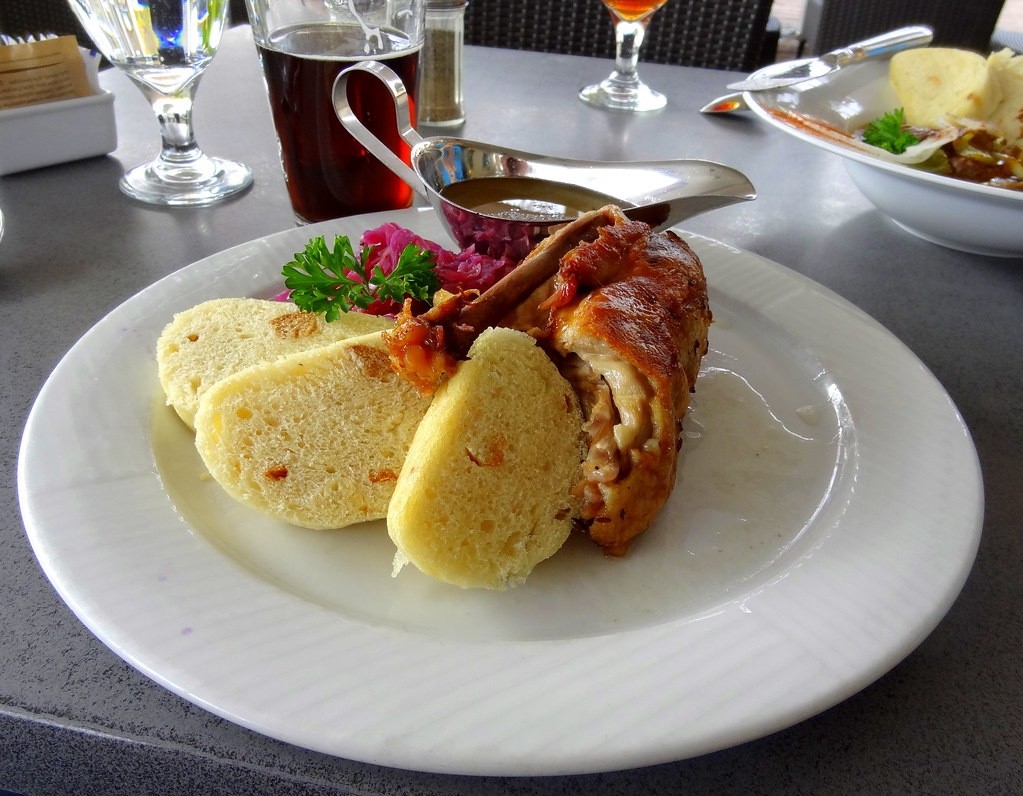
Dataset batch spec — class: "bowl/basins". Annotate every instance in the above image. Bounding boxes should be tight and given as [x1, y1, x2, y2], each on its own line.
[743, 57, 1023, 259]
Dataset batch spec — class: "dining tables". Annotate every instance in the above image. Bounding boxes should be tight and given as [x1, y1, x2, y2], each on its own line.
[0, 24, 1023, 796]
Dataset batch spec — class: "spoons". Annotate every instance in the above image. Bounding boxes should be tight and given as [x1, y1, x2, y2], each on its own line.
[700, 92, 751, 113]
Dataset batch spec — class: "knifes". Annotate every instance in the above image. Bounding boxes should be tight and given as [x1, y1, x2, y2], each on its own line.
[726, 25, 934, 91]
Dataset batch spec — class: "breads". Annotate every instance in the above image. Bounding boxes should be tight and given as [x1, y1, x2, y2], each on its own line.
[386, 323, 587, 591]
[889, 47, 1023, 140]
[154, 296, 405, 435]
[194, 327, 442, 530]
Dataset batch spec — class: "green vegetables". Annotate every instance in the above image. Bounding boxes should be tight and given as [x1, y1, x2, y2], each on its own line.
[862, 106, 918, 154]
[281, 233, 440, 322]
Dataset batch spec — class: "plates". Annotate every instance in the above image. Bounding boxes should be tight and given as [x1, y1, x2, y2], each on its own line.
[17, 206, 985, 777]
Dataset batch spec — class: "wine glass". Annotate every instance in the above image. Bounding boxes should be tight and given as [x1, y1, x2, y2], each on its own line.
[579, 0, 668, 112]
[67, 0, 254, 202]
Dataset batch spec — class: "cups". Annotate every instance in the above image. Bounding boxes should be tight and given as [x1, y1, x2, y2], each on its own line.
[247, 0, 426, 226]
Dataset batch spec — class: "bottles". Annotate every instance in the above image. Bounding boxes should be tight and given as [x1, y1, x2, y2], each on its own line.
[416, 0, 469, 138]
